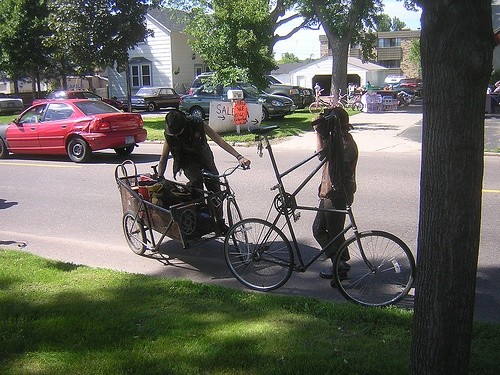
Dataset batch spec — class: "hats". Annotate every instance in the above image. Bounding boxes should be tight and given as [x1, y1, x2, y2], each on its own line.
[320, 107, 349, 123]
[166, 110, 186, 135]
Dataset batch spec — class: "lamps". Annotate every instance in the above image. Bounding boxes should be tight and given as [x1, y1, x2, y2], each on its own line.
[192, 53, 196, 60]
[173, 66, 181, 76]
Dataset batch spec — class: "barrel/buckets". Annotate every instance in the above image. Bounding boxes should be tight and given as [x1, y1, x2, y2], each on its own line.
[138, 183, 161, 202]
[151, 191, 174, 209]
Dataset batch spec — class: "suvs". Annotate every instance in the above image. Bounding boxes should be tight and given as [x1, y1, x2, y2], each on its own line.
[183, 72, 316, 110]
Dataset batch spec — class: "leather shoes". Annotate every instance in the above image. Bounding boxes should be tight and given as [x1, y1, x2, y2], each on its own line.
[331, 263, 351, 286]
[320, 265, 334, 279]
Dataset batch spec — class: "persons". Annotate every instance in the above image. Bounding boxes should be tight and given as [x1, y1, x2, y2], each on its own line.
[384, 85, 394, 90]
[494, 81, 500, 92]
[365, 81, 371, 91]
[156, 110, 251, 236]
[314, 83, 320, 96]
[312, 107, 358, 279]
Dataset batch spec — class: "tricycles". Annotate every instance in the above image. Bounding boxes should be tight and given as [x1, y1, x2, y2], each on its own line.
[114, 159, 251, 266]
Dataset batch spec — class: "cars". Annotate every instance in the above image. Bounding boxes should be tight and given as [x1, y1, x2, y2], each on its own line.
[120, 86, 184, 112]
[0, 99, 147, 164]
[31, 90, 122, 112]
[0, 91, 24, 115]
[179, 80, 298, 122]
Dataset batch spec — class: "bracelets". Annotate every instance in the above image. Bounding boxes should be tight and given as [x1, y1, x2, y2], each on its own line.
[236, 155, 244, 161]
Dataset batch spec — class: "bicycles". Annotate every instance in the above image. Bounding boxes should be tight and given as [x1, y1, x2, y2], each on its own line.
[308, 83, 344, 115]
[222, 114, 416, 309]
[337, 88, 364, 111]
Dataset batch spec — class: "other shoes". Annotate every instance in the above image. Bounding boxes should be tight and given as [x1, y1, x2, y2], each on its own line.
[217, 219, 231, 233]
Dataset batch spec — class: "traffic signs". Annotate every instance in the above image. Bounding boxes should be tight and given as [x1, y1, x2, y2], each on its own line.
[209, 101, 262, 132]
